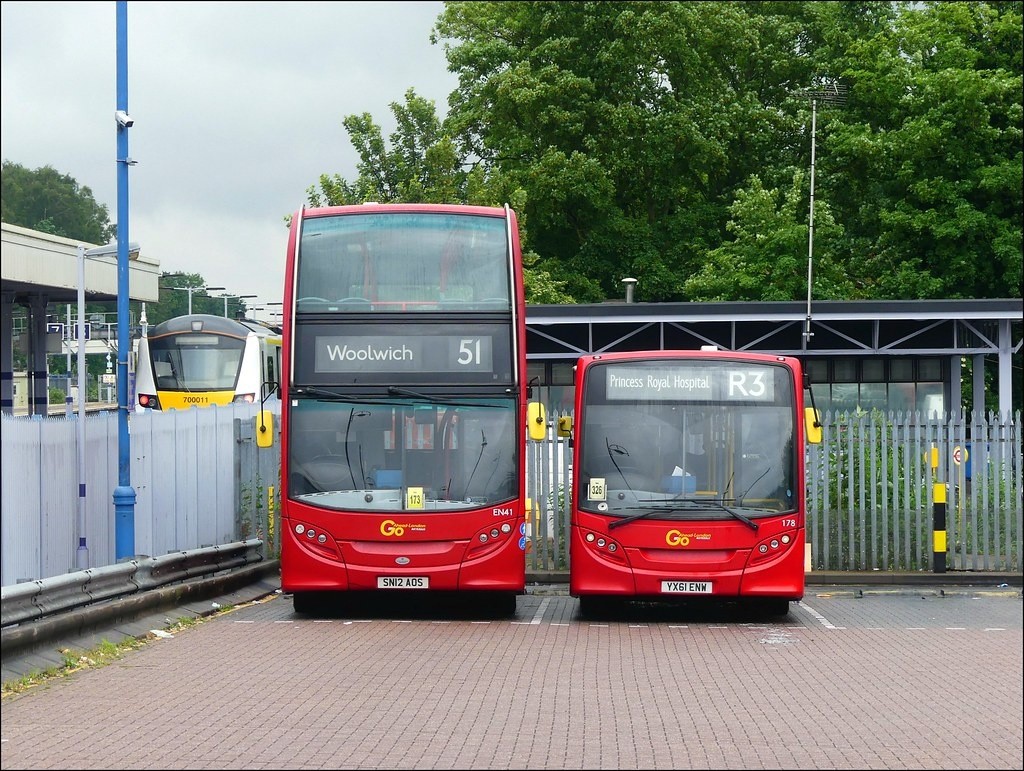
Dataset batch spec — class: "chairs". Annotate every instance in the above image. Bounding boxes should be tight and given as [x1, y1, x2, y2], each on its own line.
[440, 298, 507, 312]
[352, 443, 385, 477]
[298, 296, 370, 312]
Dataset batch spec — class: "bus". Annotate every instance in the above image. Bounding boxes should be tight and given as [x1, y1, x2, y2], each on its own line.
[258, 202, 547, 617]
[558, 343, 821, 617]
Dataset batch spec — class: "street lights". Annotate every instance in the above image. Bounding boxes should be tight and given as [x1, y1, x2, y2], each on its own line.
[78, 241, 142, 572]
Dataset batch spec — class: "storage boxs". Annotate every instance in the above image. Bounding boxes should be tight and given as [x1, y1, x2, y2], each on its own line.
[664, 476, 696, 492]
[374, 469, 401, 488]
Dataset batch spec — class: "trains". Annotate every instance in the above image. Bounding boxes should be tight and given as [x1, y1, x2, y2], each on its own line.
[134, 312, 282, 414]
